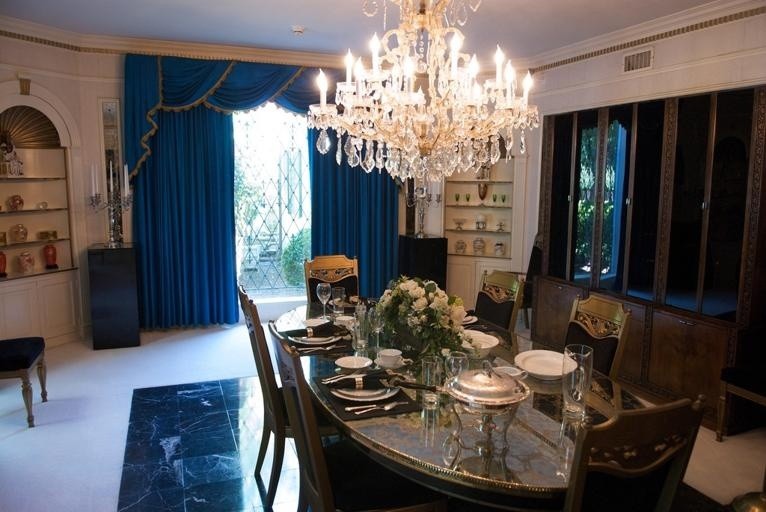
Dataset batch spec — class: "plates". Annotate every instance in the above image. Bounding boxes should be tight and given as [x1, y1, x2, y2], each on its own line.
[373, 358, 407, 368]
[288, 335, 344, 346]
[523, 376, 574, 396]
[330, 386, 400, 403]
[513, 347, 577, 382]
[461, 316, 479, 326]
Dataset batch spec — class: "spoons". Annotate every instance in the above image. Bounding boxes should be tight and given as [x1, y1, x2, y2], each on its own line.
[352, 400, 398, 418]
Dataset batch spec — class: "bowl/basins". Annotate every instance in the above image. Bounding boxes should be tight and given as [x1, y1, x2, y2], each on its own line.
[332, 368, 347, 375]
[335, 355, 372, 373]
[457, 328, 499, 361]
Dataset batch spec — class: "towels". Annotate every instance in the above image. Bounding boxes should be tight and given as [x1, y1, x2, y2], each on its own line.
[325, 373, 393, 390]
[279, 319, 339, 338]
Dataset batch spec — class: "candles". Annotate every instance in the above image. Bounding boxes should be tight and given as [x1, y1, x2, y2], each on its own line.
[89, 157, 129, 196]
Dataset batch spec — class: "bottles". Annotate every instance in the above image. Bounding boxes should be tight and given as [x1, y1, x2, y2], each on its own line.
[493, 240, 505, 256]
[10, 224, 29, 245]
[16, 252, 35, 276]
[454, 240, 466, 254]
[7, 193, 23, 210]
[472, 237, 484, 255]
[42, 243, 58, 267]
[0, 252, 6, 273]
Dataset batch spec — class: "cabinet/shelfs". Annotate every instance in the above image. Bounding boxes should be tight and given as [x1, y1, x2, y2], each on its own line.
[440, 176, 512, 260]
[446, 254, 511, 311]
[0, 269, 78, 352]
[581, 291, 647, 400]
[539, 106, 602, 292]
[530, 275, 586, 354]
[646, 301, 765, 437]
[659, 86, 765, 330]
[595, 99, 668, 304]
[0, 148, 76, 280]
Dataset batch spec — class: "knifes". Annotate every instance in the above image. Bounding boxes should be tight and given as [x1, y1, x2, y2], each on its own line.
[342, 401, 409, 412]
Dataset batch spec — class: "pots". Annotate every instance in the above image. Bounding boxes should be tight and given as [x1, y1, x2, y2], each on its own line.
[454, 456, 517, 484]
[394, 359, 531, 416]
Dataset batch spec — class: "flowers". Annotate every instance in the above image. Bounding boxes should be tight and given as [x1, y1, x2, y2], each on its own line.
[373, 274, 483, 359]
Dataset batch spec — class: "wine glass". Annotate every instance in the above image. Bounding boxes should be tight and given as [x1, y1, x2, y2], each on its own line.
[367, 306, 386, 351]
[454, 193, 460, 207]
[493, 194, 498, 207]
[477, 183, 487, 208]
[315, 282, 332, 320]
[465, 193, 470, 207]
[500, 193, 506, 208]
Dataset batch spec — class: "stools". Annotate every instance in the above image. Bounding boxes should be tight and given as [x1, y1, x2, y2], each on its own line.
[0, 336, 49, 428]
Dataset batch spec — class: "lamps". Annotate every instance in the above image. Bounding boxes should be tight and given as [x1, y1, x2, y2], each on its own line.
[305, 0, 542, 200]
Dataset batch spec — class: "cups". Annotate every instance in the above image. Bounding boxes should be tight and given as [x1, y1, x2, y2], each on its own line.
[330, 287, 369, 353]
[445, 351, 470, 377]
[562, 343, 595, 419]
[374, 348, 404, 368]
[555, 416, 585, 482]
[418, 410, 440, 449]
[494, 367, 527, 383]
[420, 356, 443, 412]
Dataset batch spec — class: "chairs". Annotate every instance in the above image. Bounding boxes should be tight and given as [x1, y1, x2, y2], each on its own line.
[474, 270, 525, 333]
[562, 291, 633, 381]
[714, 362, 765, 443]
[234, 281, 338, 512]
[268, 318, 450, 512]
[559, 393, 707, 512]
[302, 254, 360, 319]
